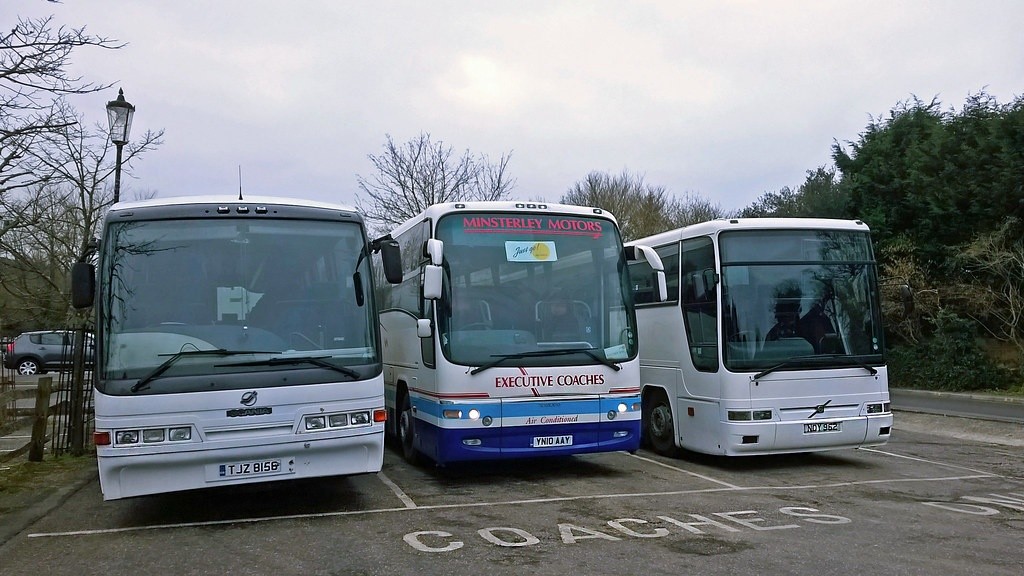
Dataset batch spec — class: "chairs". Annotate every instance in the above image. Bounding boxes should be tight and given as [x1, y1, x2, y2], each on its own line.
[299, 282, 341, 331]
[449, 299, 592, 341]
[635, 283, 842, 344]
[146, 274, 183, 308]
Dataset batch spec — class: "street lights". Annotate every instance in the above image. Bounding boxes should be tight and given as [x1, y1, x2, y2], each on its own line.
[104, 87, 137, 204]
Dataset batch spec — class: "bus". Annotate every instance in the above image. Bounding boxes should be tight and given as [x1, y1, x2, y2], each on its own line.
[327, 199, 667, 476]
[470, 216, 914, 458]
[69, 191, 406, 504]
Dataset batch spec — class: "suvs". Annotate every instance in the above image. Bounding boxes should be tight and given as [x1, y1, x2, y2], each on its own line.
[2, 330, 96, 376]
